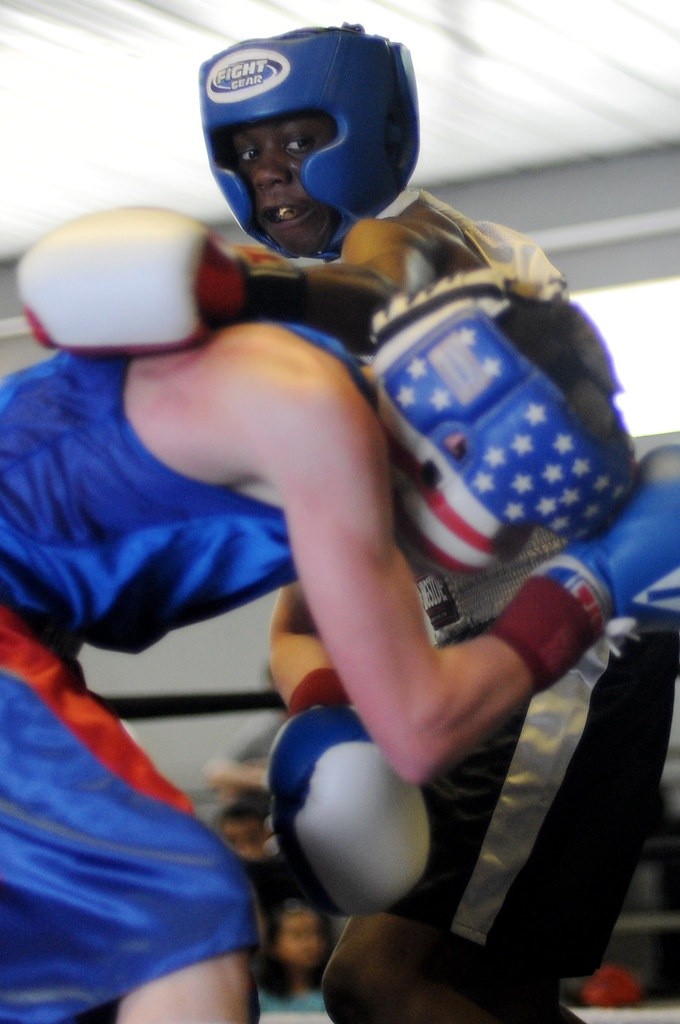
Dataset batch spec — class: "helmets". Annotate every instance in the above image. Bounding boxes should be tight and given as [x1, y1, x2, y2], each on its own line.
[197, 22, 421, 261]
[360, 264, 637, 581]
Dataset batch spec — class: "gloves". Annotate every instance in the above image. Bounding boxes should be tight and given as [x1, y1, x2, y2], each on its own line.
[533, 444, 680, 632]
[16, 207, 304, 359]
[260, 702, 431, 919]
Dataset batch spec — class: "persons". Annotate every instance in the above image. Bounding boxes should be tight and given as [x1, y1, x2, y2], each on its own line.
[17, 21, 680, 1024]
[0, 266, 680, 1024]
